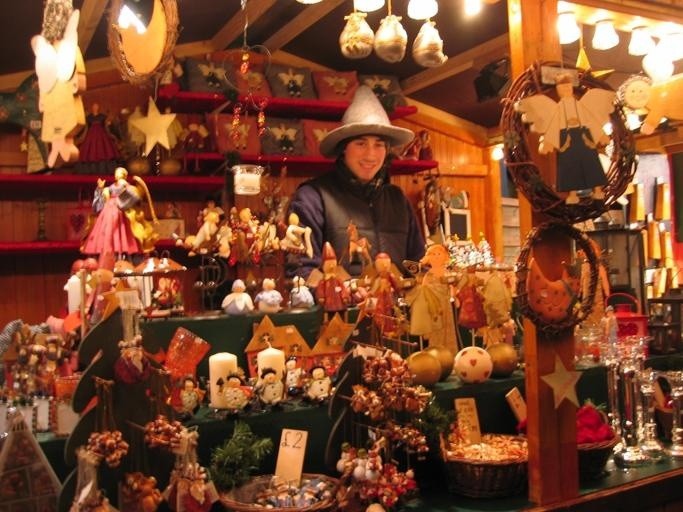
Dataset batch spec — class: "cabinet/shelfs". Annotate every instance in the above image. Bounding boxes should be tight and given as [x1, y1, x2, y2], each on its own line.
[0, 92, 683, 512]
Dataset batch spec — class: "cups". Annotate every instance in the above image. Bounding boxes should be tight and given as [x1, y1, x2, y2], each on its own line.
[575, 329, 605, 366]
[165, 327, 212, 375]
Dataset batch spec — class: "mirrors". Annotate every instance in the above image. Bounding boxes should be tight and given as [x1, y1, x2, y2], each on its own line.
[106, 1, 179, 87]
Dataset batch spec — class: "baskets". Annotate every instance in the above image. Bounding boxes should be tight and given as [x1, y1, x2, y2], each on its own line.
[438, 434, 530, 500]
[576, 433, 622, 478]
[217, 473, 340, 512]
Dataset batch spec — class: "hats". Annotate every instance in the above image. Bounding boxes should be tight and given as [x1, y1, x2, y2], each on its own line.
[318, 84, 415, 158]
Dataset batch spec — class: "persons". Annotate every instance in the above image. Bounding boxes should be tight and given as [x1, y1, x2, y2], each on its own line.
[286, 87, 427, 284]
[78, 166, 142, 253]
[46, 86, 517, 433]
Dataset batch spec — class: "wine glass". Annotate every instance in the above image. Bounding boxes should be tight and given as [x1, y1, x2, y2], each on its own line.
[606, 336, 683, 467]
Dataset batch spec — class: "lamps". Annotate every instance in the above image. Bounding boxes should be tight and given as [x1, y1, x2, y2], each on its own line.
[555, 0, 656, 57]
